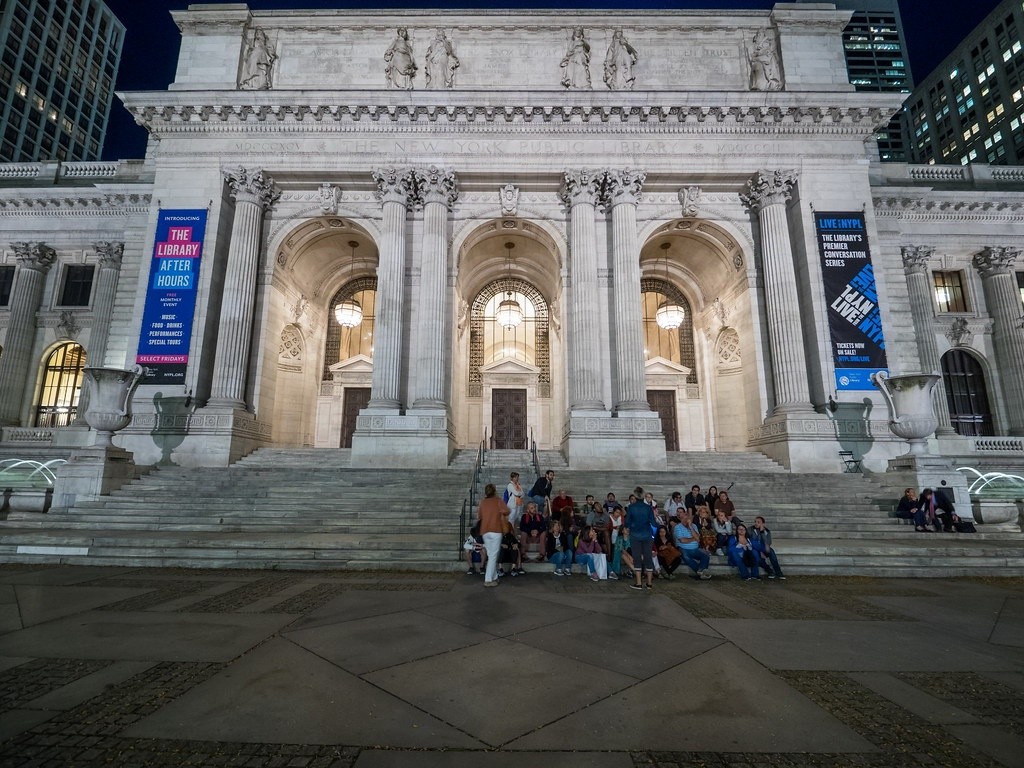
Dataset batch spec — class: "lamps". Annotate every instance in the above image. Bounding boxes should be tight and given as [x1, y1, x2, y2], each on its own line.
[334, 241, 363, 329]
[495, 242, 524, 330]
[656, 242, 684, 331]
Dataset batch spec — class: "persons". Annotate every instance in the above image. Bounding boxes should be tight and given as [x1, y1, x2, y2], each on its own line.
[424, 26, 460, 90]
[561, 24, 592, 91]
[603, 26, 638, 90]
[383, 27, 419, 89]
[463, 471, 787, 590]
[896, 488, 960, 533]
[748, 28, 784, 91]
[240, 25, 278, 89]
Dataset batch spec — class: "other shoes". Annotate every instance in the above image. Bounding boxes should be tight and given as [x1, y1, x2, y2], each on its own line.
[914, 525, 933, 533]
[716, 548, 724, 556]
[645, 583, 652, 589]
[511, 569, 518, 576]
[497, 568, 504, 576]
[742, 576, 752, 582]
[467, 567, 473, 574]
[935, 526, 942, 533]
[943, 527, 956, 533]
[697, 569, 712, 579]
[608, 572, 618, 579]
[768, 573, 775, 578]
[706, 550, 711, 556]
[657, 573, 664, 578]
[518, 568, 525, 573]
[554, 568, 564, 575]
[564, 568, 571, 575]
[629, 584, 642, 589]
[484, 580, 498, 586]
[480, 567, 485, 574]
[777, 573, 786, 579]
[590, 572, 599, 581]
[751, 575, 761, 581]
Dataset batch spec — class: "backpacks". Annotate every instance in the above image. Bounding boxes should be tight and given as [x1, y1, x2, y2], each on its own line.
[503, 486, 512, 505]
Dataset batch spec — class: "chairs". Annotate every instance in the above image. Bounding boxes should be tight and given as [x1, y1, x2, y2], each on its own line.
[840, 451, 863, 473]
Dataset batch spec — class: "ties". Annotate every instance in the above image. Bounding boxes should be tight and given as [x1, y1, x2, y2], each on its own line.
[929, 497, 934, 519]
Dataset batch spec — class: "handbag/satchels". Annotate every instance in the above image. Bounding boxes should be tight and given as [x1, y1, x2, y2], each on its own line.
[497, 498, 509, 534]
[658, 542, 681, 565]
[956, 522, 976, 533]
[586, 552, 607, 579]
